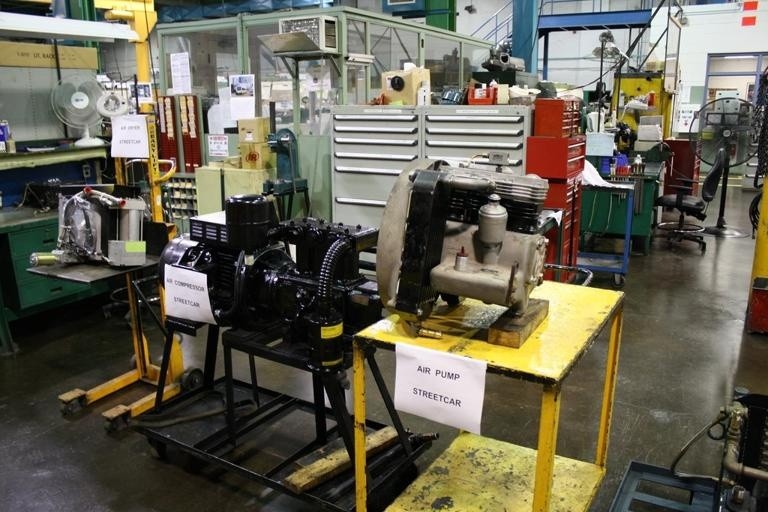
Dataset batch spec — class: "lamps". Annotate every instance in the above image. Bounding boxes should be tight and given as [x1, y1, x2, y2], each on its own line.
[621, 100, 648, 121]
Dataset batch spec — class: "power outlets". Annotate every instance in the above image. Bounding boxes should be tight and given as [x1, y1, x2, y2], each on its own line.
[83, 164, 90, 177]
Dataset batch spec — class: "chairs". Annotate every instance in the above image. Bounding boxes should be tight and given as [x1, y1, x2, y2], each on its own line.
[657, 148, 725, 251]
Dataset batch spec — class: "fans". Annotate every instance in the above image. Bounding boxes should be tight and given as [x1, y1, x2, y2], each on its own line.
[688, 98, 763, 238]
[51, 72, 105, 146]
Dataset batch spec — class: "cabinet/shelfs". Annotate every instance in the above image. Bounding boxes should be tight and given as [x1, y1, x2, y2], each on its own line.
[533, 99, 580, 138]
[527, 135, 586, 178]
[664, 138, 703, 196]
[544, 178, 582, 282]
[0, 219, 109, 317]
[354, 276, 626, 512]
[576, 181, 635, 286]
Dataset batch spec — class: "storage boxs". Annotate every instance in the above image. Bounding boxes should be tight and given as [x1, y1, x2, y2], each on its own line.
[383, 69, 431, 105]
[238, 118, 271, 143]
[240, 143, 277, 169]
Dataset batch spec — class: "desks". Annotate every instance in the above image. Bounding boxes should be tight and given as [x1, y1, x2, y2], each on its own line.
[580, 161, 666, 255]
[0, 149, 108, 184]
[634, 139, 661, 161]
[194, 166, 275, 216]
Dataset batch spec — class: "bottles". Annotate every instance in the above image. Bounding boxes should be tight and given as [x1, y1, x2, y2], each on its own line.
[647, 90, 655, 107]
[618, 90, 625, 107]
[634, 154, 643, 175]
